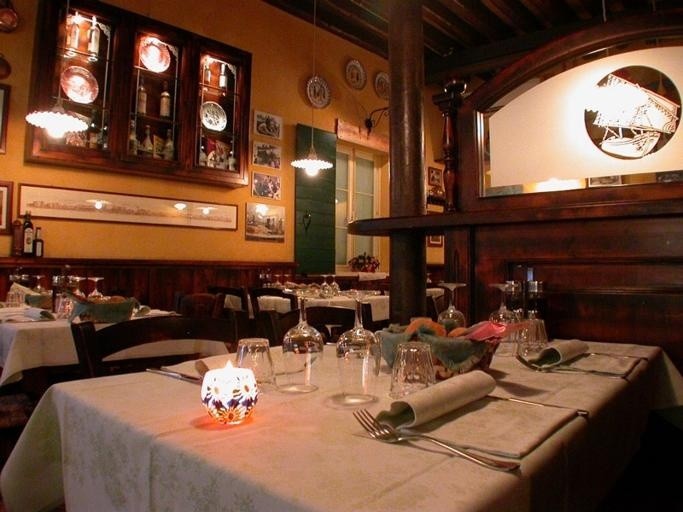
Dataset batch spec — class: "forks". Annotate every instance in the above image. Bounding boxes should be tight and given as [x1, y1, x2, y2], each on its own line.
[352, 409, 521, 472]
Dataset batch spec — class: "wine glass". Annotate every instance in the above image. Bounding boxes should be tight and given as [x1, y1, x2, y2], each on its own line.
[274, 275, 340, 298]
[283, 283, 519, 404]
[33, 276, 103, 300]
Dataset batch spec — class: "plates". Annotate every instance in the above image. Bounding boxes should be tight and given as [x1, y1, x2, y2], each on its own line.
[307, 59, 391, 108]
[59, 37, 228, 160]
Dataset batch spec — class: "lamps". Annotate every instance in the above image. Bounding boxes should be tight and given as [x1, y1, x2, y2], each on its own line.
[25, 0, 93, 138]
[201, 359, 259, 425]
[289, 0, 334, 177]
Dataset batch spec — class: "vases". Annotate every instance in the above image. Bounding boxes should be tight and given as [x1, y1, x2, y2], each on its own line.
[352, 266, 375, 273]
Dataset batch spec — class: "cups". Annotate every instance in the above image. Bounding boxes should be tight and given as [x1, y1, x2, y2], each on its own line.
[515, 320, 548, 357]
[237, 337, 277, 397]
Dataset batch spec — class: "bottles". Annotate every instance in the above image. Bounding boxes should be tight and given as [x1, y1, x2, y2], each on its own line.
[499, 281, 545, 321]
[34, 227, 44, 258]
[266, 268, 272, 288]
[259, 266, 265, 287]
[52, 276, 62, 313]
[22, 210, 34, 257]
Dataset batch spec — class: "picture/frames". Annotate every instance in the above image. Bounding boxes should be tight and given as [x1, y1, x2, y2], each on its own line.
[1, 180, 14, 236]
[17, 183, 238, 231]
[427, 167, 442, 188]
[252, 141, 281, 170]
[251, 171, 282, 201]
[0, 83, 12, 155]
[426, 210, 444, 248]
[252, 109, 283, 140]
[245, 201, 286, 239]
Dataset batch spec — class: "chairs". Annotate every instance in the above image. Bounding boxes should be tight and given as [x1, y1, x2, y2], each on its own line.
[208, 285, 249, 328]
[21, 309, 200, 418]
[334, 275, 359, 291]
[426, 297, 438, 322]
[360, 303, 390, 332]
[375, 280, 391, 291]
[301, 276, 325, 321]
[305, 306, 356, 344]
[250, 288, 298, 343]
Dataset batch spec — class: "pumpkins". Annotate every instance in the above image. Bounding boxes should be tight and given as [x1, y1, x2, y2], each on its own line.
[404, 318, 447, 338]
[447, 326, 468, 337]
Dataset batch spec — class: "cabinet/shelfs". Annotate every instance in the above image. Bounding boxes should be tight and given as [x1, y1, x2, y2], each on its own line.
[22, 0, 254, 190]
[349, 196, 683, 346]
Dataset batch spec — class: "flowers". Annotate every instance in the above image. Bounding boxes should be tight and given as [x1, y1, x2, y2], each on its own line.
[349, 252, 380, 271]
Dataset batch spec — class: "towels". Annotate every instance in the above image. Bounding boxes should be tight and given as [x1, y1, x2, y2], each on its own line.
[1, 303, 58, 323]
[372, 370, 577, 458]
[528, 339, 639, 377]
